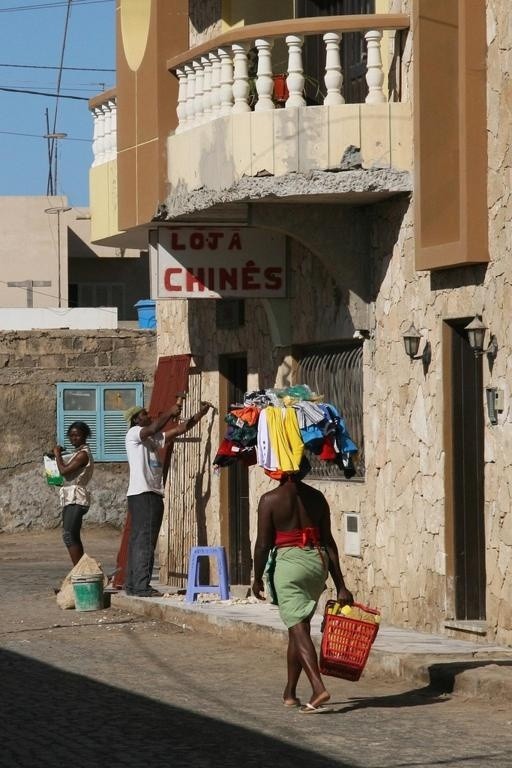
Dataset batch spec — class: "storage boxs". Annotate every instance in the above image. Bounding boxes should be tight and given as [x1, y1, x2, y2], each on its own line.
[43, 454, 72, 487]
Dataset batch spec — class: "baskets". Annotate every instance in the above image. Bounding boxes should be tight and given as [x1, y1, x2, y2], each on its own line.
[318, 599, 382, 682]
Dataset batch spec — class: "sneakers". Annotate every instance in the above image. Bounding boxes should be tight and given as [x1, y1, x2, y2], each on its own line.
[126, 586, 164, 598]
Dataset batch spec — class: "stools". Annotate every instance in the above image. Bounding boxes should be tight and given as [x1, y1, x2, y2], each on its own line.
[185, 545, 230, 603]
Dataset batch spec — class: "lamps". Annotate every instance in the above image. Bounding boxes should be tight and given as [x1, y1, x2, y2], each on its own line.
[402, 322, 431, 366]
[465, 317, 498, 361]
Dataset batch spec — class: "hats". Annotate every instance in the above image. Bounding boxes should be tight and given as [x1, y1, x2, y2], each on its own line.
[123, 405, 146, 428]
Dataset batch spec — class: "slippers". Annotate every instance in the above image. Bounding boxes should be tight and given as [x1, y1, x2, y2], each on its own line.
[282, 696, 333, 714]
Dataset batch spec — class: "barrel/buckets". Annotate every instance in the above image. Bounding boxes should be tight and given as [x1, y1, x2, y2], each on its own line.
[43, 450, 71, 486]
[72, 574, 104, 611]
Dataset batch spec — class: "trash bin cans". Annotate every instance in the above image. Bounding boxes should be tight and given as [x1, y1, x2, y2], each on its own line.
[134, 299, 156, 329]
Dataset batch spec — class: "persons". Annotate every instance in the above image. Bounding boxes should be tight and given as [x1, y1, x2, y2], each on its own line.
[252, 457, 355, 717]
[53, 420, 95, 566]
[121, 398, 214, 598]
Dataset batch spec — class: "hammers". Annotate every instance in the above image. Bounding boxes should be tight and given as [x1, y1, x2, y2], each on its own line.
[173, 391, 188, 423]
